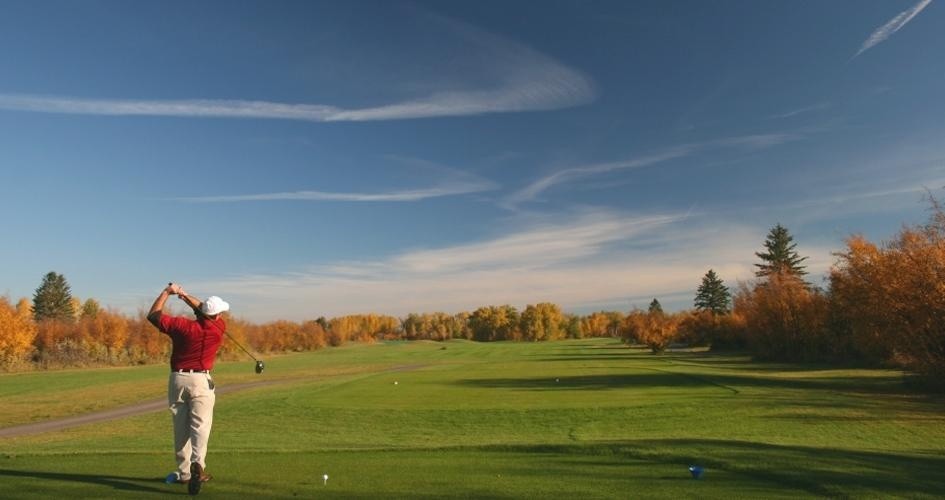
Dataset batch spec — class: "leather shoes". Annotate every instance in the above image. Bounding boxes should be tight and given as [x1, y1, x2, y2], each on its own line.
[188, 462, 202, 495]
[181, 473, 212, 484]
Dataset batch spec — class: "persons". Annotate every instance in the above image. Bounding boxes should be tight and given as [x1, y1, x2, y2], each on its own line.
[146, 284, 229, 494]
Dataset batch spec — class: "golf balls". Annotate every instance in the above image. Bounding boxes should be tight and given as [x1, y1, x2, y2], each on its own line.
[555, 378, 560, 383]
[394, 381, 398, 385]
[322, 474, 328, 480]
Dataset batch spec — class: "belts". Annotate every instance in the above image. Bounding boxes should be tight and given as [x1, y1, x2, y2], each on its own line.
[172, 369, 210, 374]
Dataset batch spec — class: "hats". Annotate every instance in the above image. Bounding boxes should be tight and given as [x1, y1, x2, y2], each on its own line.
[201, 295, 230, 317]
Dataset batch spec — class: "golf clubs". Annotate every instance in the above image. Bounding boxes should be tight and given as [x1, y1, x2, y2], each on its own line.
[168, 283, 264, 373]
